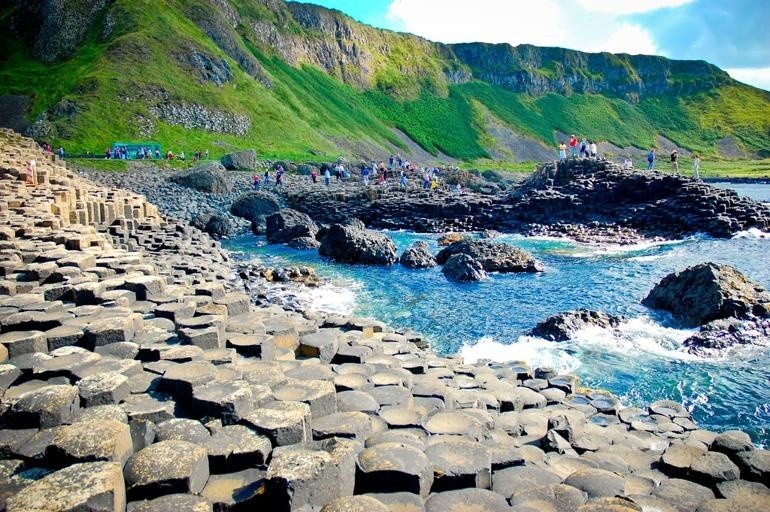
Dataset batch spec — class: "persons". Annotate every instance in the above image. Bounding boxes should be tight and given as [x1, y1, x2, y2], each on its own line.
[647, 149, 655, 169]
[693, 155, 699, 179]
[251, 152, 466, 196]
[41, 143, 210, 162]
[558, 134, 608, 162]
[624, 158, 633, 170]
[670, 150, 679, 175]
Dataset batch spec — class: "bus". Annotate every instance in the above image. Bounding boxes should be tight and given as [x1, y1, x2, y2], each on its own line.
[110, 142, 163, 160]
